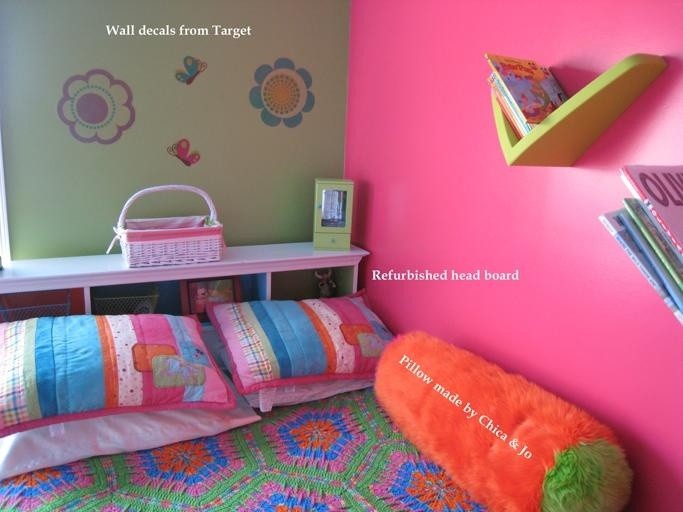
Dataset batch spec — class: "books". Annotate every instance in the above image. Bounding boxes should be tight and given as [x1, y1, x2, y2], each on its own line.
[484, 51, 568, 141]
[598, 164, 682, 327]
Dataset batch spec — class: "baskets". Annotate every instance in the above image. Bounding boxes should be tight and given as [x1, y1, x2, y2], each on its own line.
[108, 184, 224, 267]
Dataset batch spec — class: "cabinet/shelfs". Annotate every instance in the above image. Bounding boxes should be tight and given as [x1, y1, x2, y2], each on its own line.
[0, 242, 371, 319]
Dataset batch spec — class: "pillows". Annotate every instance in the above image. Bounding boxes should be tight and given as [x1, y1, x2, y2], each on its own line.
[205, 288, 396, 396]
[375, 330, 634, 512]
[217, 347, 374, 412]
[0, 312, 237, 436]
[0, 371, 262, 483]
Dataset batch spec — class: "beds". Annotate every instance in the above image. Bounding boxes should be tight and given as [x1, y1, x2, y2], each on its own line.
[1, 367, 493, 510]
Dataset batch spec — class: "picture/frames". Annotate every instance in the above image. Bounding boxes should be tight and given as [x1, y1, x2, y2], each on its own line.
[178, 275, 241, 321]
